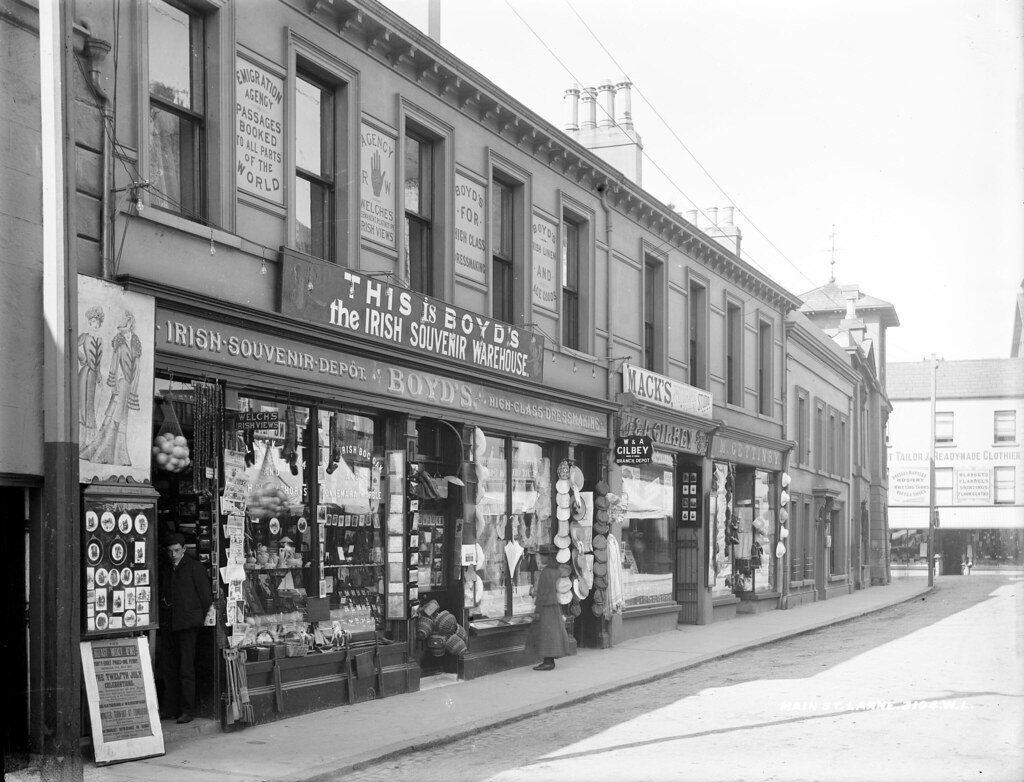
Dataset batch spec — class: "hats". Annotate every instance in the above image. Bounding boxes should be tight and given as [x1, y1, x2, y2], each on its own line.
[592, 481, 609, 616]
[776, 473, 791, 558]
[535, 544, 557, 554]
[555, 459, 589, 619]
[464, 428, 485, 604]
[163, 533, 185, 545]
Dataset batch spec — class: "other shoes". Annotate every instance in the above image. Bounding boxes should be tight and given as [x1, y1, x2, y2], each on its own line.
[177, 713, 194, 723]
[533, 664, 555, 670]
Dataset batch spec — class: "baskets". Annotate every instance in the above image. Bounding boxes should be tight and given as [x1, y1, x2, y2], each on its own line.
[416, 608, 468, 657]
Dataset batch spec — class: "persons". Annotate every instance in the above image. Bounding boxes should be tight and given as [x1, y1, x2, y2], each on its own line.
[158, 533, 213, 723]
[525, 544, 570, 671]
